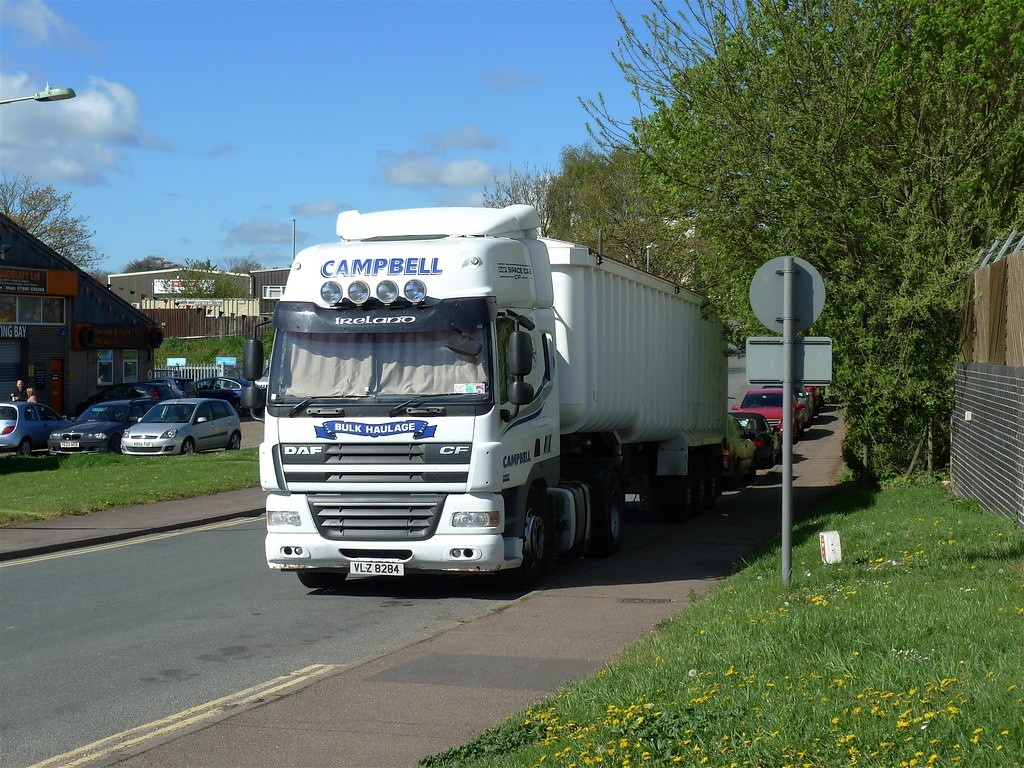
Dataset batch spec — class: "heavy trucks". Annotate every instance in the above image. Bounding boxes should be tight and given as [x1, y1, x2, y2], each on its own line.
[242, 204, 731, 596]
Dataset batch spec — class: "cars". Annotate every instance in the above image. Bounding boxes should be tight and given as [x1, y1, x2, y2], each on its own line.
[730, 389, 805, 444]
[722, 415, 758, 487]
[135, 377, 200, 399]
[762, 385, 815, 428]
[46, 396, 160, 455]
[195, 377, 264, 417]
[0, 402, 75, 456]
[727, 412, 780, 466]
[72, 382, 177, 418]
[120, 398, 241, 457]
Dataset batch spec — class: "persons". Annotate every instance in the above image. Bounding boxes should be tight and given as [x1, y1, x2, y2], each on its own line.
[10, 380, 27, 401]
[26, 388, 36, 403]
